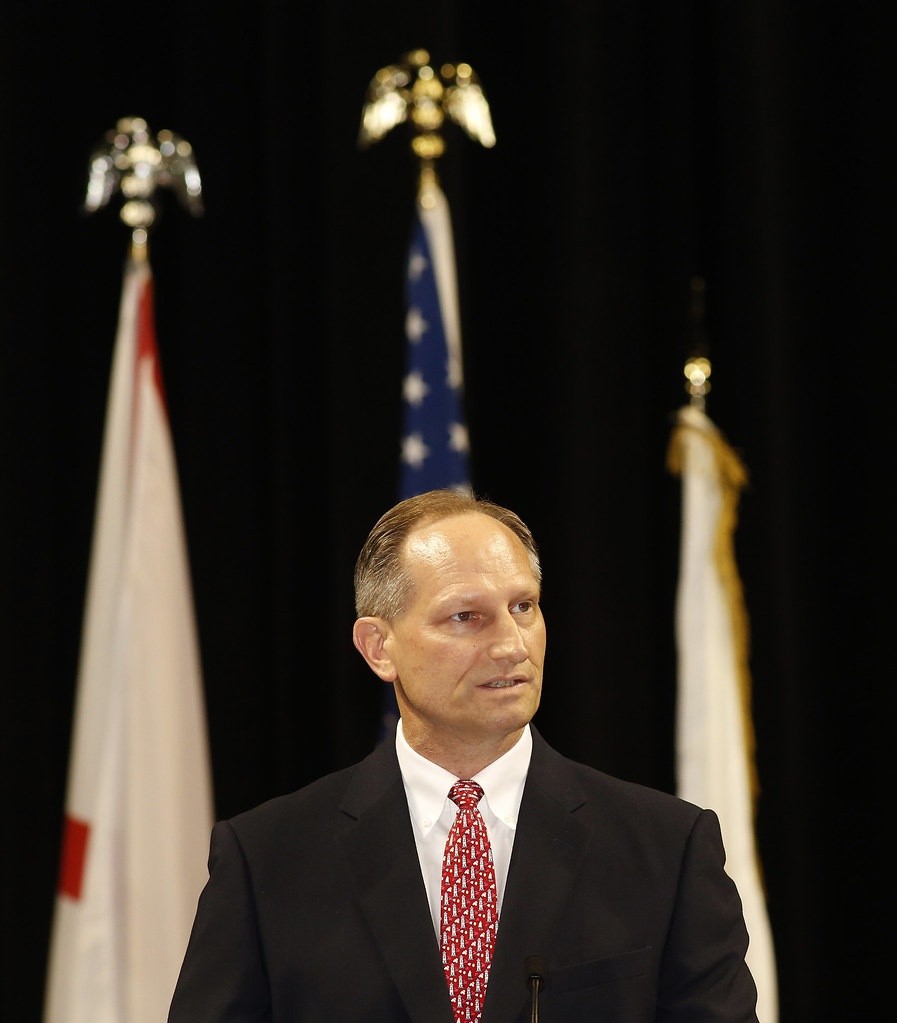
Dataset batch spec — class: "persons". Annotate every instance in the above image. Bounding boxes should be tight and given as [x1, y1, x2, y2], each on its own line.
[165, 492, 759, 1023]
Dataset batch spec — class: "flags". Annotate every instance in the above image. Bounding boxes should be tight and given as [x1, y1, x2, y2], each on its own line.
[48, 229, 215, 1023]
[402, 192, 470, 496]
[667, 415, 778, 1023]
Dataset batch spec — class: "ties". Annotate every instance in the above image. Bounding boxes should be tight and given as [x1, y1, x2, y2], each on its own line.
[437, 776, 501, 1023]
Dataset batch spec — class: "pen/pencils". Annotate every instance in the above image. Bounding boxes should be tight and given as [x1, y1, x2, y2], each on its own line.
[531, 976, 541, 1023]
